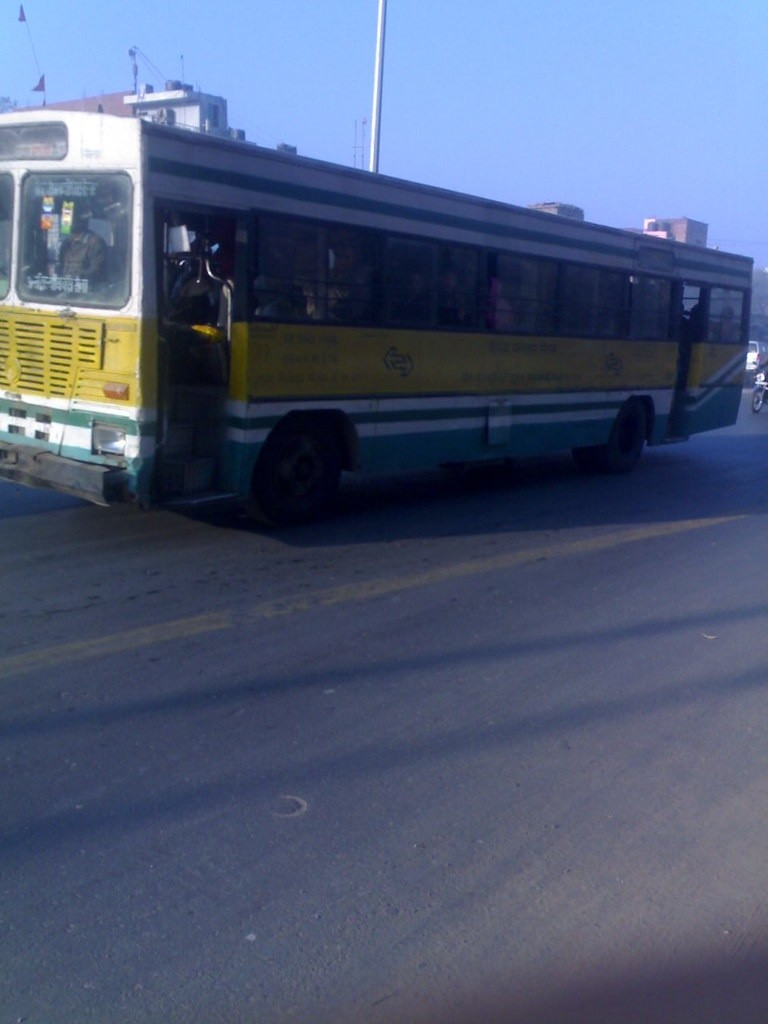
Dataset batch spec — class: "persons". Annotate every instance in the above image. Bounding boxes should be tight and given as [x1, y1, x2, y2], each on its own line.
[757, 359, 768, 371]
[268, 268, 740, 346]
[58, 212, 105, 283]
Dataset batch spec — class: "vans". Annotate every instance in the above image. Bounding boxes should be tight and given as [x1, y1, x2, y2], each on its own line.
[746, 340, 768, 371]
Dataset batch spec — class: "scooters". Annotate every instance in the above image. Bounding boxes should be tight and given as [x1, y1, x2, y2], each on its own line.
[751, 368, 768, 413]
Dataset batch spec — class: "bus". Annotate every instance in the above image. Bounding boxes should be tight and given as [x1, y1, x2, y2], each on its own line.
[1, 109, 753, 528]
